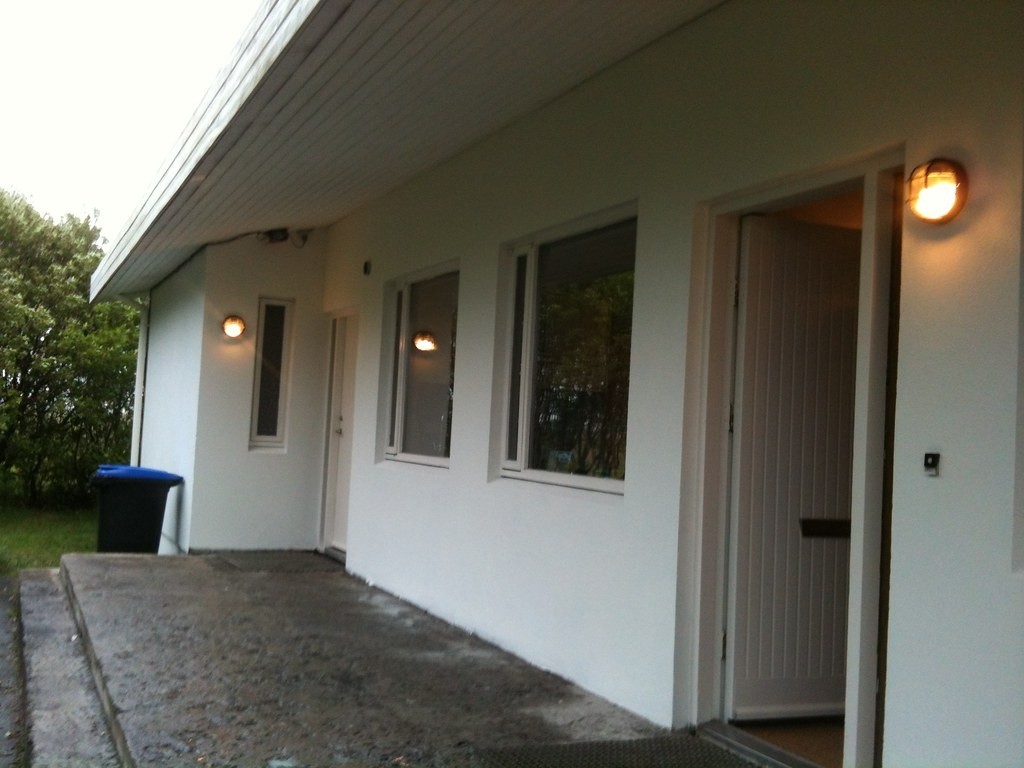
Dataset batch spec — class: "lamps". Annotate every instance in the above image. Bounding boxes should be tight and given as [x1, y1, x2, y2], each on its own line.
[221, 315, 246, 338]
[412, 330, 439, 353]
[903, 156, 969, 226]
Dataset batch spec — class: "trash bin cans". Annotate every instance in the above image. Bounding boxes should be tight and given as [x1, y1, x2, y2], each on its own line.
[86, 464, 183, 554]
